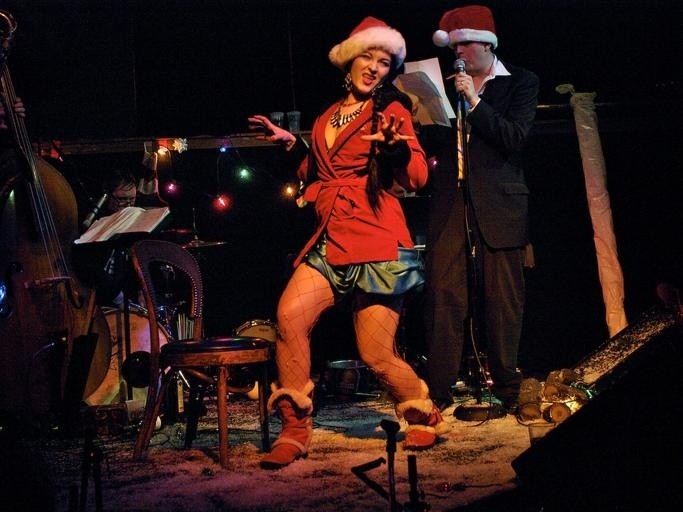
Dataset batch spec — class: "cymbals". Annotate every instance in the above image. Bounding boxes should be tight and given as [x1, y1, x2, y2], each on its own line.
[177, 239, 226, 248]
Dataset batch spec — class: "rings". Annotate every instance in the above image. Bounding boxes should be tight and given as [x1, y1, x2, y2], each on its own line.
[460, 81, 464, 86]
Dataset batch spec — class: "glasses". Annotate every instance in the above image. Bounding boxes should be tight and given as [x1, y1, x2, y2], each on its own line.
[113, 197, 137, 208]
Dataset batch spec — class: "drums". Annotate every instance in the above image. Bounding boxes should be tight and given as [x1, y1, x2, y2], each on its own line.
[85, 306, 171, 410]
[232, 319, 276, 340]
[329, 359, 375, 398]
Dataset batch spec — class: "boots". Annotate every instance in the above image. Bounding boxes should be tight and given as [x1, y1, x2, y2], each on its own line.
[259, 380, 316, 469]
[394, 379, 443, 450]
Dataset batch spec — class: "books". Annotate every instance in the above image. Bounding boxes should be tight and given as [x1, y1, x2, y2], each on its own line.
[73, 205, 170, 244]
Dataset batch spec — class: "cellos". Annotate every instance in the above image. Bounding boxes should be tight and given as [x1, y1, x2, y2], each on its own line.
[0, 23, 112, 416]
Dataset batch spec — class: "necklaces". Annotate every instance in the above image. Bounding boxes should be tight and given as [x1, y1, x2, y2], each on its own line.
[330, 99, 366, 127]
[342, 101, 361, 108]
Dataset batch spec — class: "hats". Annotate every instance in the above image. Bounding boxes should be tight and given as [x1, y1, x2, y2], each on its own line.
[434, 6, 498, 48]
[330, 17, 406, 68]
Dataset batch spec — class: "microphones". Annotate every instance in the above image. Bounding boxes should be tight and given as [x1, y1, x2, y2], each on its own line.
[83, 189, 108, 232]
[452, 57, 465, 100]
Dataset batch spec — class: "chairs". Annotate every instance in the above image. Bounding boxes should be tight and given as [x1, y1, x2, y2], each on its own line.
[131, 239, 270, 468]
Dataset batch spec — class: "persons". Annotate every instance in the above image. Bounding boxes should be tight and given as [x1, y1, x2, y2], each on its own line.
[412, 5, 539, 413]
[0, 90, 27, 131]
[246, 16, 452, 467]
[70, 168, 147, 310]
